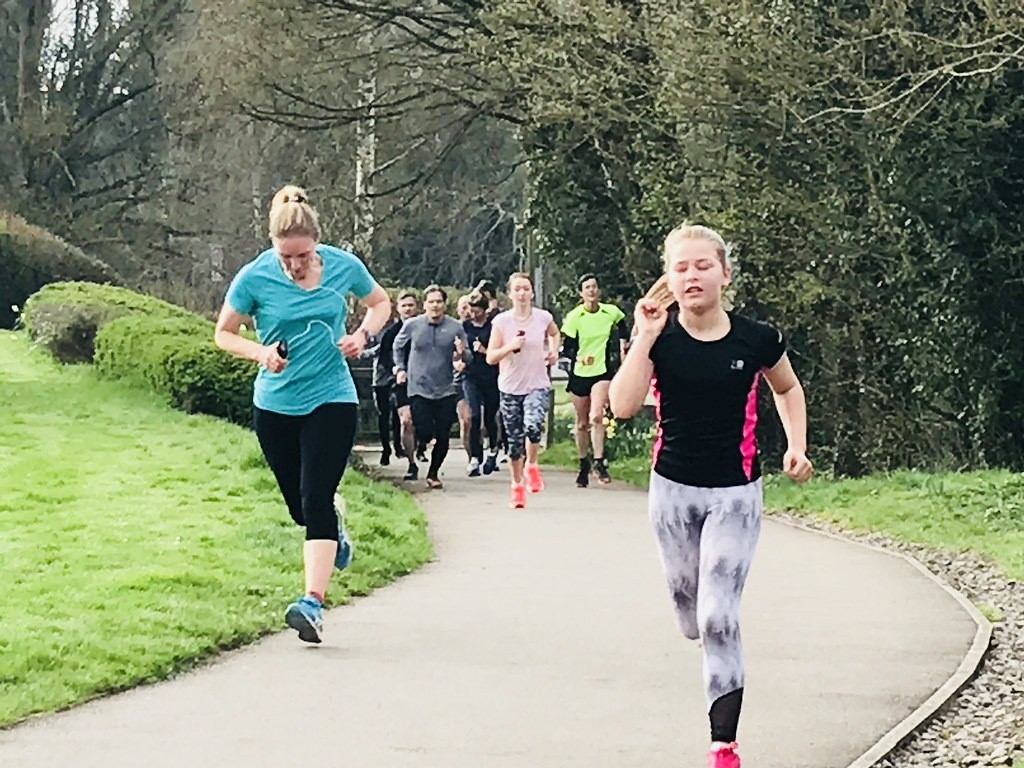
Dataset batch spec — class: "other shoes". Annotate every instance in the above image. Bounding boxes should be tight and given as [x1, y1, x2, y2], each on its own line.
[396, 450, 406, 458]
[416, 454, 429, 462]
[707, 741, 741, 768]
[381, 447, 392, 466]
[467, 453, 499, 476]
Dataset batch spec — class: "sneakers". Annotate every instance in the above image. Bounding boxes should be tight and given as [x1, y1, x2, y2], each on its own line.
[404, 465, 419, 479]
[284, 595, 323, 643]
[426, 477, 442, 488]
[593, 463, 612, 483]
[511, 480, 526, 508]
[523, 466, 543, 493]
[334, 493, 352, 571]
[576, 460, 591, 487]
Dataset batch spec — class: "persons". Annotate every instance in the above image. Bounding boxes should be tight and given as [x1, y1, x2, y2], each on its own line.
[357, 281, 507, 479]
[487, 272, 559, 508]
[562, 272, 627, 487]
[393, 285, 473, 489]
[215, 186, 391, 644]
[608, 224, 813, 768]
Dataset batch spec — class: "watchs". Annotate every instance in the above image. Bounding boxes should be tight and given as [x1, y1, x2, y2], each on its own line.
[357, 328, 377, 348]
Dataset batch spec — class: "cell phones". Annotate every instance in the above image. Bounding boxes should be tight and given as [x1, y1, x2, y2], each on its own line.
[276, 336, 288, 360]
